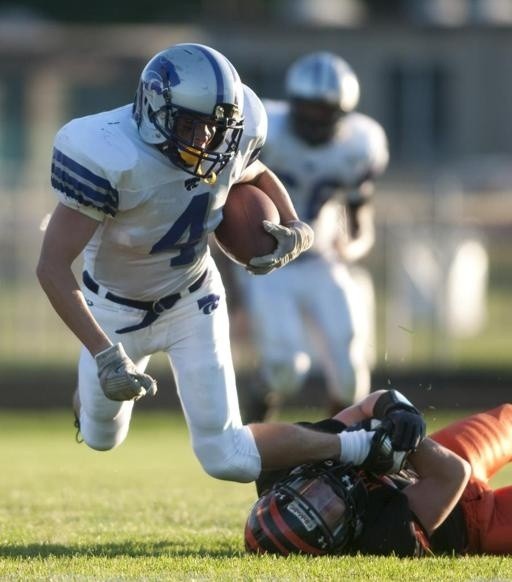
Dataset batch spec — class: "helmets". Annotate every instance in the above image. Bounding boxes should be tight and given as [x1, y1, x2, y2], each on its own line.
[130, 42, 244, 183]
[245, 458, 370, 557]
[285, 50, 361, 146]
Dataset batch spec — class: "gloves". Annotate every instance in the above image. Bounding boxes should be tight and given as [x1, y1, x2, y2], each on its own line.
[94, 342, 158, 402]
[383, 407, 426, 453]
[243, 219, 315, 276]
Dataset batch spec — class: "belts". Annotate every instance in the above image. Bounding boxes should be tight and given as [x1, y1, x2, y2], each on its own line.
[82, 268, 208, 335]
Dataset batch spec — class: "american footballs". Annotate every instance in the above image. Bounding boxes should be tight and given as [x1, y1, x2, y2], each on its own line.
[214, 184, 282, 265]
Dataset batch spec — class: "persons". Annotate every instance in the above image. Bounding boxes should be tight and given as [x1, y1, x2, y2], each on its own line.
[35, 44, 410, 483]
[235, 50, 391, 427]
[245, 388, 512, 555]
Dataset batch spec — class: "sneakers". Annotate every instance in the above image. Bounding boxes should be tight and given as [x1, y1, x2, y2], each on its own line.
[343, 418, 418, 491]
